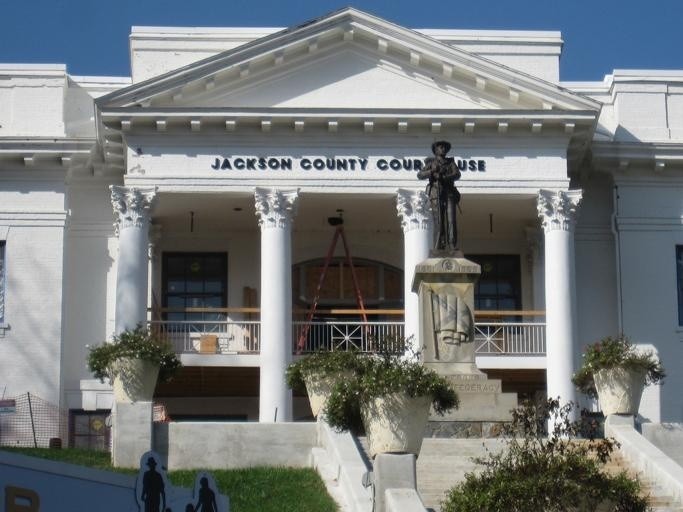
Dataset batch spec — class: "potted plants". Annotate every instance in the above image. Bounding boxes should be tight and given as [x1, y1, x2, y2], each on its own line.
[571, 334, 667, 417]
[283, 331, 461, 458]
[83, 320, 182, 402]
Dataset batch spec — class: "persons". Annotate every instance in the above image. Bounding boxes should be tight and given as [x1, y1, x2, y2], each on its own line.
[139, 456, 165, 512]
[192, 476, 217, 512]
[416, 140, 461, 250]
